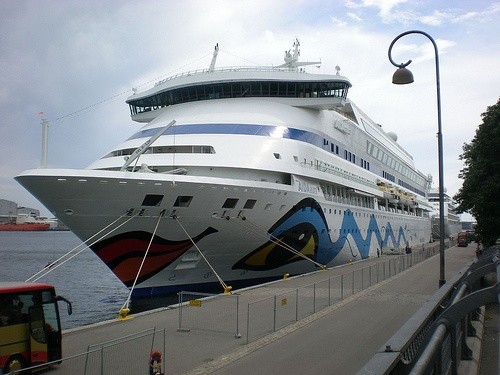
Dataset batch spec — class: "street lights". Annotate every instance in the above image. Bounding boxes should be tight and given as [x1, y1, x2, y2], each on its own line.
[389, 31, 448, 290]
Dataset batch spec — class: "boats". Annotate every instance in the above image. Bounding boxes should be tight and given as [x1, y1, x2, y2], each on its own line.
[427, 187, 460, 235]
[15, 40, 434, 301]
[0, 219, 50, 231]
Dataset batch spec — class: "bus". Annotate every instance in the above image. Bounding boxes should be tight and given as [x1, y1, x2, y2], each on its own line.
[1, 282, 72, 374]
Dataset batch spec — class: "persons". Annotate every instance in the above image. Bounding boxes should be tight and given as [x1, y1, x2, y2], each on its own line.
[405, 246, 407, 254]
[423, 244, 425, 251]
[17, 301, 24, 316]
[376, 247, 381, 257]
